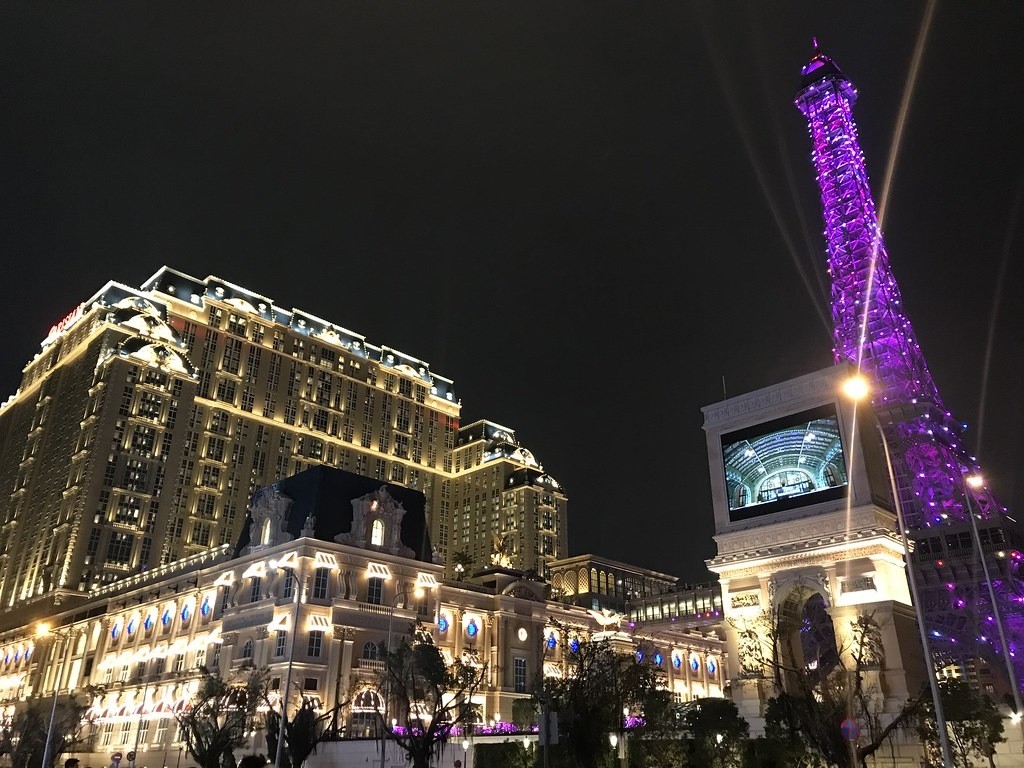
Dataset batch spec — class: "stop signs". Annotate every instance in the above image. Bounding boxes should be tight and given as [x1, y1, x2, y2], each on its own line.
[114, 751, 123, 761]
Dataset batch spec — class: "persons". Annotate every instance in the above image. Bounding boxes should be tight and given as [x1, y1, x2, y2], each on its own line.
[65, 758, 80, 768]
[237, 755, 266, 768]
[305, 512, 316, 529]
[668, 582, 704, 593]
[432, 543, 444, 564]
[841, 577, 874, 593]
[808, 572, 833, 601]
[363, 483, 395, 515]
[768, 579, 785, 604]
[731, 594, 760, 608]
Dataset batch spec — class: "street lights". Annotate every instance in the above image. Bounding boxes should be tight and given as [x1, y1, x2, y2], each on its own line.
[37, 621, 70, 768]
[963, 471, 1024, 725]
[267, 558, 302, 768]
[842, 372, 955, 768]
[380, 590, 425, 768]
